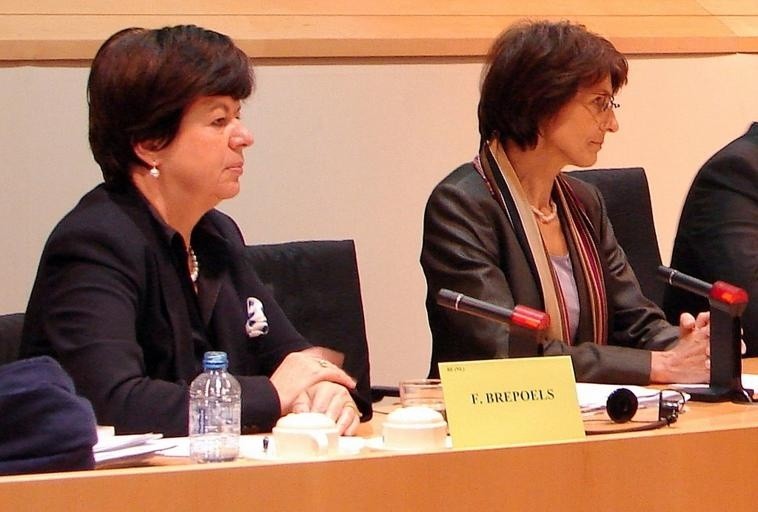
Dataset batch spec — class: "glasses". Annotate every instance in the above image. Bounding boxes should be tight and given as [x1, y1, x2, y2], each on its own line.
[573, 89, 621, 112]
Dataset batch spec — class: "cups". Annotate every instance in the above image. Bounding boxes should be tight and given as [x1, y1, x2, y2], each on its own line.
[399, 378, 447, 427]
[270, 413, 338, 466]
[383, 406, 448, 454]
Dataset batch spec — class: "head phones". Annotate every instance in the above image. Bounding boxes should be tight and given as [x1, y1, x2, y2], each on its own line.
[580, 388, 680, 435]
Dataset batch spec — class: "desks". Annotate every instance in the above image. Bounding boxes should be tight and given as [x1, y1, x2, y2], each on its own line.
[0, 358, 758, 512]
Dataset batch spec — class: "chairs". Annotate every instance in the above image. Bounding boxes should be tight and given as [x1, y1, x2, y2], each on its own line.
[0, 311, 26, 366]
[243, 238, 370, 396]
[560, 166, 669, 311]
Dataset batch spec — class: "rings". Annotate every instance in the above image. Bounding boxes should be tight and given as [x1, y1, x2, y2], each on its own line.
[346, 403, 360, 416]
[319, 358, 328, 369]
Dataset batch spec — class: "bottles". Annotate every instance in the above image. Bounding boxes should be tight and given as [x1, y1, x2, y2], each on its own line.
[187, 352, 241, 467]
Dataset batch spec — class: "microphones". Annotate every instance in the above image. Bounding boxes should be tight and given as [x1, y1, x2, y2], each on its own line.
[436, 288, 550, 359]
[654, 265, 753, 403]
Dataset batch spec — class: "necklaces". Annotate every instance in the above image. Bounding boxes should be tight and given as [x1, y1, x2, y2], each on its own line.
[189, 246, 202, 285]
[527, 198, 561, 225]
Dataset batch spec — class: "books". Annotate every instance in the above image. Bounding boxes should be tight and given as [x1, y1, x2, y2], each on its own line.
[575, 380, 690, 417]
[92, 420, 180, 468]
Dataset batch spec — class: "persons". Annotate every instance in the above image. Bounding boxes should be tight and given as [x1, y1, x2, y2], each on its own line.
[418, 14, 747, 387]
[17, 22, 365, 443]
[662, 124, 758, 360]
